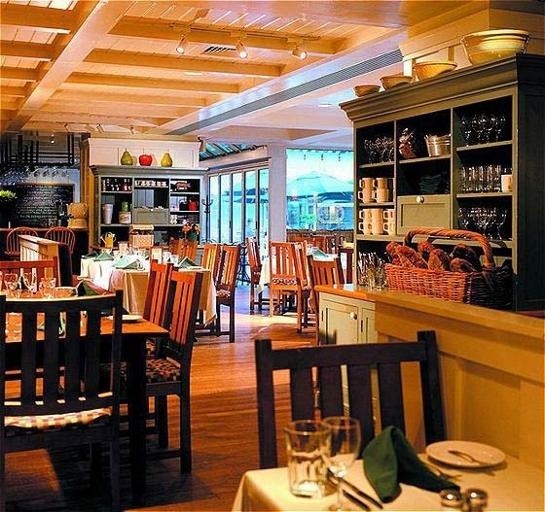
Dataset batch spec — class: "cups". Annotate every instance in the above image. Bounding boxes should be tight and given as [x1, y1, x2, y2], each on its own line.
[364, 137, 394, 163]
[458, 165, 513, 194]
[358, 178, 393, 204]
[134, 181, 166, 187]
[284, 418, 326, 497]
[102, 242, 178, 271]
[358, 209, 395, 236]
[170, 215, 178, 224]
[121, 202, 128, 211]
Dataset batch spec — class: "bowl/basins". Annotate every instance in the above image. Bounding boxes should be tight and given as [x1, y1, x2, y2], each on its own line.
[380, 76, 413, 91]
[459, 31, 530, 64]
[412, 62, 457, 80]
[354, 84, 381, 97]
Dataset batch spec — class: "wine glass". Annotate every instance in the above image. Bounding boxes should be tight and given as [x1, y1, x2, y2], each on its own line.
[0, 270, 78, 333]
[457, 208, 507, 239]
[321, 415, 361, 511]
[460, 113, 506, 146]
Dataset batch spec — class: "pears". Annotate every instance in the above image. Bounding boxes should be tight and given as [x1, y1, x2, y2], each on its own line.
[120, 148, 134, 164]
[160, 152, 173, 167]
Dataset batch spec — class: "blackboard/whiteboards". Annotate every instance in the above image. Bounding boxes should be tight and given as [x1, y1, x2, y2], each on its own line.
[0, 182, 77, 228]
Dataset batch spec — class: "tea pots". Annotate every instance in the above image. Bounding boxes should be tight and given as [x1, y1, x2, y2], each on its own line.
[100, 232, 117, 248]
[188, 200, 198, 211]
[178, 199, 188, 210]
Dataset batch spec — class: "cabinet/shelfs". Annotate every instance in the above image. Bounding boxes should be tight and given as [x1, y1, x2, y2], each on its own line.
[339, 52, 544, 313]
[315, 288, 378, 417]
[88, 163, 207, 265]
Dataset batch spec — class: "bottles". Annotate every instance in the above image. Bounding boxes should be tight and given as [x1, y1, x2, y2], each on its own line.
[465, 486, 489, 511]
[358, 249, 392, 289]
[102, 178, 131, 191]
[439, 489, 464, 511]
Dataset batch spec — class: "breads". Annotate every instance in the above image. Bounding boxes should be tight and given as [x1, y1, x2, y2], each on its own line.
[386, 241, 481, 274]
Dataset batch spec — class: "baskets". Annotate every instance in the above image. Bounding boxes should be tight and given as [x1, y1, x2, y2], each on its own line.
[385, 228, 513, 310]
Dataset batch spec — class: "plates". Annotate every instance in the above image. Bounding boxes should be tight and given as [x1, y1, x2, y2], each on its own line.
[109, 313, 143, 322]
[420, 171, 450, 194]
[427, 439, 506, 468]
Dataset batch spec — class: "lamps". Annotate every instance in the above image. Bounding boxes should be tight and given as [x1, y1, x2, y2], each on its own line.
[171, 24, 320, 60]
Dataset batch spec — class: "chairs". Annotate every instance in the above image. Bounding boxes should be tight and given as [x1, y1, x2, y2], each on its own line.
[109, 268, 203, 474]
[44, 227, 75, 257]
[142, 259, 170, 322]
[5, 227, 38, 261]
[2, 257, 59, 291]
[0, 287, 124, 512]
[246, 228, 354, 334]
[169, 238, 243, 342]
[253, 330, 447, 472]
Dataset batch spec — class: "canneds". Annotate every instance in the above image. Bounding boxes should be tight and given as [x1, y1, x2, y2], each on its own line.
[121, 201, 129, 212]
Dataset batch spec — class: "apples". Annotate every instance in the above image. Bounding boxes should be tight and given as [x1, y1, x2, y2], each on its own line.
[139, 155, 153, 166]
